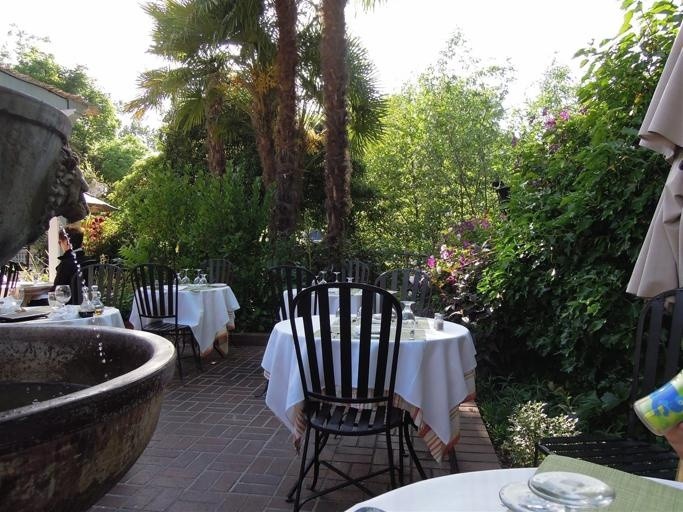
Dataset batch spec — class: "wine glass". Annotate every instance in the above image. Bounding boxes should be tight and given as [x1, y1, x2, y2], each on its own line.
[528, 471, 616, 512]
[399, 300, 415, 320]
[499, 481, 576, 512]
[174, 268, 208, 291]
[10, 289, 25, 311]
[311, 270, 354, 287]
[48, 284, 104, 319]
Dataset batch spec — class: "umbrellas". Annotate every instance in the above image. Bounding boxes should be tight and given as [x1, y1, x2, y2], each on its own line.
[625, 18, 683, 304]
[81, 193, 118, 216]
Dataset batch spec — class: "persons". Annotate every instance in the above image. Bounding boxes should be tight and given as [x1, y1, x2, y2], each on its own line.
[632, 363, 683, 484]
[45, 226, 90, 305]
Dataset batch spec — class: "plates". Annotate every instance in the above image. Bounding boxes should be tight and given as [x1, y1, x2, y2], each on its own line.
[208, 284, 226, 287]
[354, 313, 391, 339]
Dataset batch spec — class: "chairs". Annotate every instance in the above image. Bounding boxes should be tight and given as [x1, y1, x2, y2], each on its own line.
[0, 257, 241, 384]
[534, 288, 683, 480]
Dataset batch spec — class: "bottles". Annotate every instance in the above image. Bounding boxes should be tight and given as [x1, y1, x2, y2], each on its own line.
[433, 313, 443, 330]
